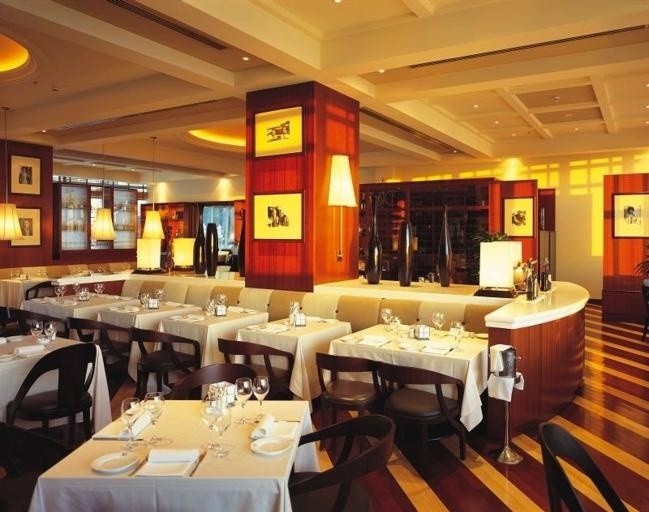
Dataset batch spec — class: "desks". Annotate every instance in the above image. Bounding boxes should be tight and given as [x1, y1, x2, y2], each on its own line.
[1, 334, 96, 444]
[331, 324, 489, 448]
[33, 399, 310, 510]
[217, 250, 230, 265]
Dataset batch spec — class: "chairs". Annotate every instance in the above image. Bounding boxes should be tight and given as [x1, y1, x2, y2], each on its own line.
[641, 284, 649, 340]
[3, 269, 494, 361]
[375, 358, 466, 469]
[4, 343, 98, 470]
[314, 351, 385, 467]
[537, 419, 629, 511]
[168, 364, 261, 400]
[290, 414, 396, 512]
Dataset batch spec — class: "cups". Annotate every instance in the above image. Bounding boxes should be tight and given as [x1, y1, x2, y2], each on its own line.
[20, 273, 31, 280]
[136, 287, 164, 309]
[414, 273, 440, 288]
[205, 290, 229, 318]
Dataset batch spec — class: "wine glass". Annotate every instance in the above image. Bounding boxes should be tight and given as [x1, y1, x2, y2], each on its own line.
[56, 282, 105, 304]
[29, 319, 57, 346]
[120, 393, 146, 449]
[199, 376, 270, 457]
[381, 307, 464, 345]
[142, 391, 167, 446]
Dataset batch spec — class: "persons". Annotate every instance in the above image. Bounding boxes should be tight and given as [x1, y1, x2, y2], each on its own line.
[21, 220, 32, 236]
[19, 167, 28, 184]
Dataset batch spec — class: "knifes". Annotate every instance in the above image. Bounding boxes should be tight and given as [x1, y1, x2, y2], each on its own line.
[188, 451, 207, 477]
[129, 454, 148, 477]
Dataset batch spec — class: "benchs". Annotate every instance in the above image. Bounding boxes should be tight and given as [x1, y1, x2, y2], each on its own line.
[0, 262, 133, 281]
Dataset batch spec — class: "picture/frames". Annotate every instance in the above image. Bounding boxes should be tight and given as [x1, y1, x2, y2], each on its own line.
[612, 191, 649, 239]
[502, 196, 534, 238]
[251, 192, 304, 244]
[9, 154, 42, 197]
[8, 207, 43, 248]
[253, 104, 304, 159]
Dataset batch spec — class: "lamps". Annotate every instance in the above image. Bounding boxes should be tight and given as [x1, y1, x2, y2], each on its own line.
[91, 143, 118, 240]
[132, 238, 166, 275]
[327, 153, 360, 261]
[141, 136, 166, 240]
[472, 239, 524, 300]
[172, 236, 197, 271]
[0, 106, 26, 242]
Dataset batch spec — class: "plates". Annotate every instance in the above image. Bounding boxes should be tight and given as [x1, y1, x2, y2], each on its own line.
[249, 436, 295, 456]
[90, 452, 139, 474]
[168, 315, 204, 323]
[111, 305, 140, 314]
[474, 332, 489, 339]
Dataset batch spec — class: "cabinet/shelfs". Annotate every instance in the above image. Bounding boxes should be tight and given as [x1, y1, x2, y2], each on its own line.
[360, 188, 488, 256]
[54, 183, 91, 251]
[90, 186, 113, 250]
[114, 185, 148, 249]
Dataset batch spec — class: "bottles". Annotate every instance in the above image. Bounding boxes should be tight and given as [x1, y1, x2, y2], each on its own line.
[295, 313, 305, 325]
[512, 258, 551, 300]
[237, 207, 245, 276]
[364, 195, 383, 283]
[192, 216, 218, 277]
[435, 203, 454, 287]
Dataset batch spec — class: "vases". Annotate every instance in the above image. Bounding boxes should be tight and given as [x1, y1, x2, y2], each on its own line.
[193, 224, 207, 274]
[205, 222, 219, 277]
[437, 200, 453, 287]
[366, 194, 386, 285]
[237, 207, 246, 277]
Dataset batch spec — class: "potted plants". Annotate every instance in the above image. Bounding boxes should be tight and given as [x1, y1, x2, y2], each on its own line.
[633, 244, 649, 286]
